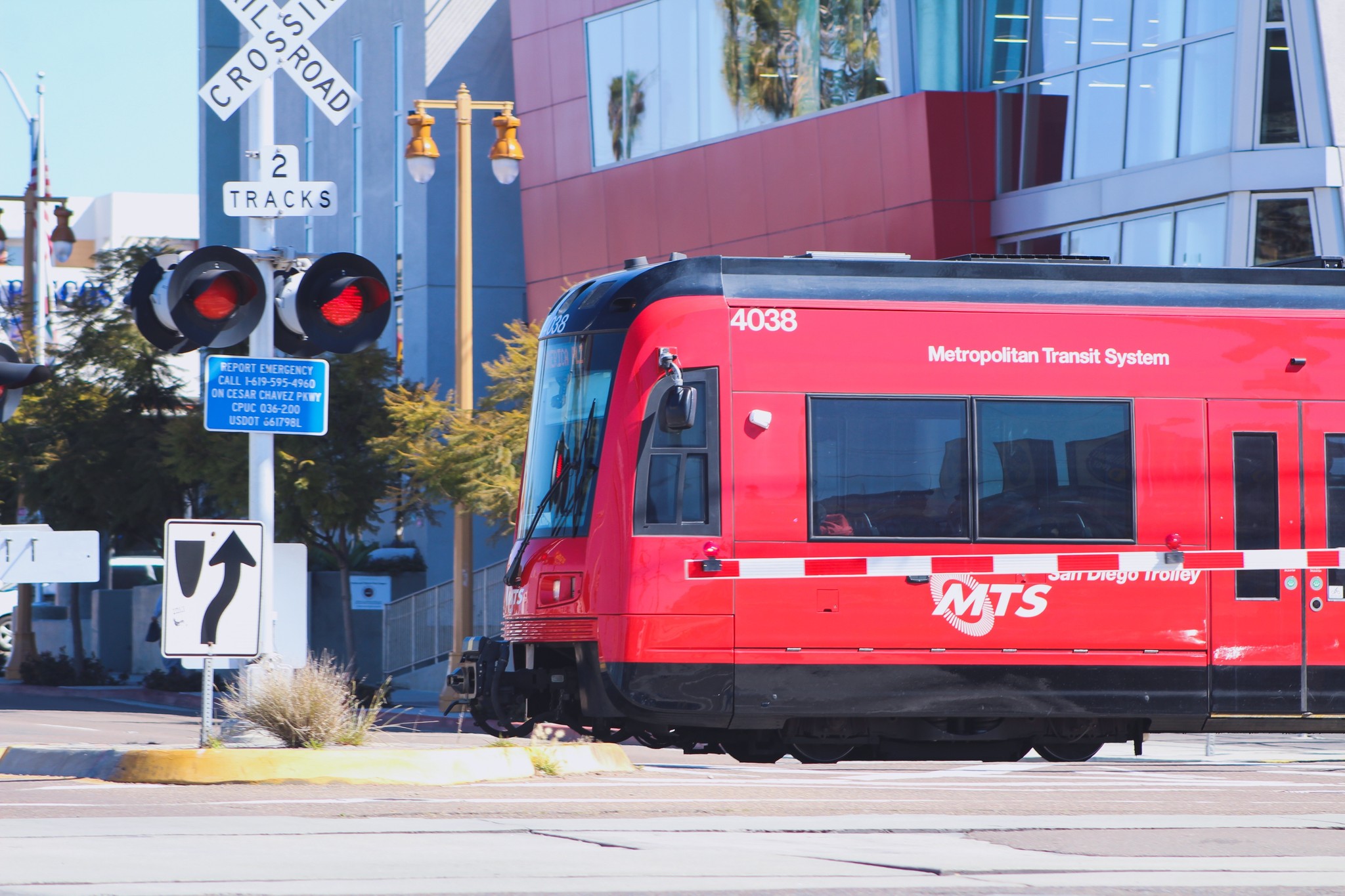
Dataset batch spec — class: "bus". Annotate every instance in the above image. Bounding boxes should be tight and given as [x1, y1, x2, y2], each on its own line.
[441, 249, 1345, 765]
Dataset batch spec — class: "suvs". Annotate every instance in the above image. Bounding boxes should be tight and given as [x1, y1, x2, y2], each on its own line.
[0, 555, 169, 652]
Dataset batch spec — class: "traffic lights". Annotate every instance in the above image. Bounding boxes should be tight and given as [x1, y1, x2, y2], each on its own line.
[0, 342, 50, 423]
[168, 245, 266, 348]
[277, 252, 391, 354]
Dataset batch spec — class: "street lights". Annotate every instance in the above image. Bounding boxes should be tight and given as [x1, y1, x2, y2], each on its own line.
[0, 184, 76, 681]
[406, 81, 525, 713]
